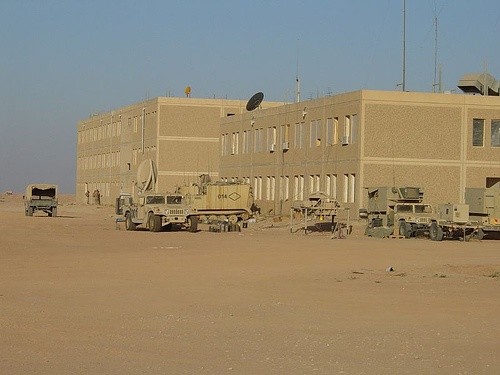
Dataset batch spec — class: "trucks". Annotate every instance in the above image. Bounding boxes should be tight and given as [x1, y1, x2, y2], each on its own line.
[23, 183, 59, 217]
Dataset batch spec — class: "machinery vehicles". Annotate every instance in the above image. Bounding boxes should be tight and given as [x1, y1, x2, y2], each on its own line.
[366, 186, 436, 238]
[173, 174, 260, 233]
[431, 181, 500, 241]
[115, 160, 198, 233]
